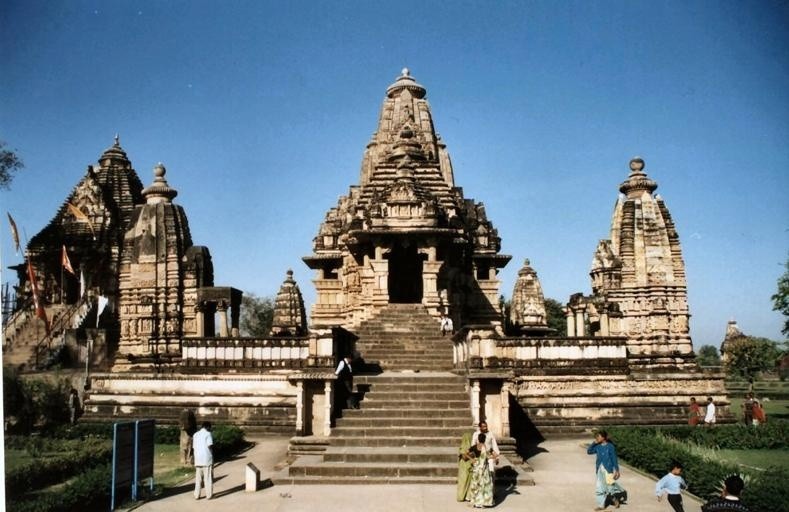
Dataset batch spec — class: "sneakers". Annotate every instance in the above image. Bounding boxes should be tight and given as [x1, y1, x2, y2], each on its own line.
[613, 495, 620, 509]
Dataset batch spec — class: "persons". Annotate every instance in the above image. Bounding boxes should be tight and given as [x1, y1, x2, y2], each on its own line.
[68, 389, 76, 425]
[743, 391, 767, 426]
[178, 409, 197, 464]
[688, 397, 702, 424]
[457, 430, 475, 502]
[333, 353, 360, 410]
[440, 313, 447, 336]
[192, 421, 217, 499]
[701, 475, 756, 512]
[463, 434, 497, 507]
[586, 430, 627, 511]
[656, 460, 690, 512]
[343, 357, 358, 409]
[470, 420, 500, 499]
[704, 397, 717, 425]
